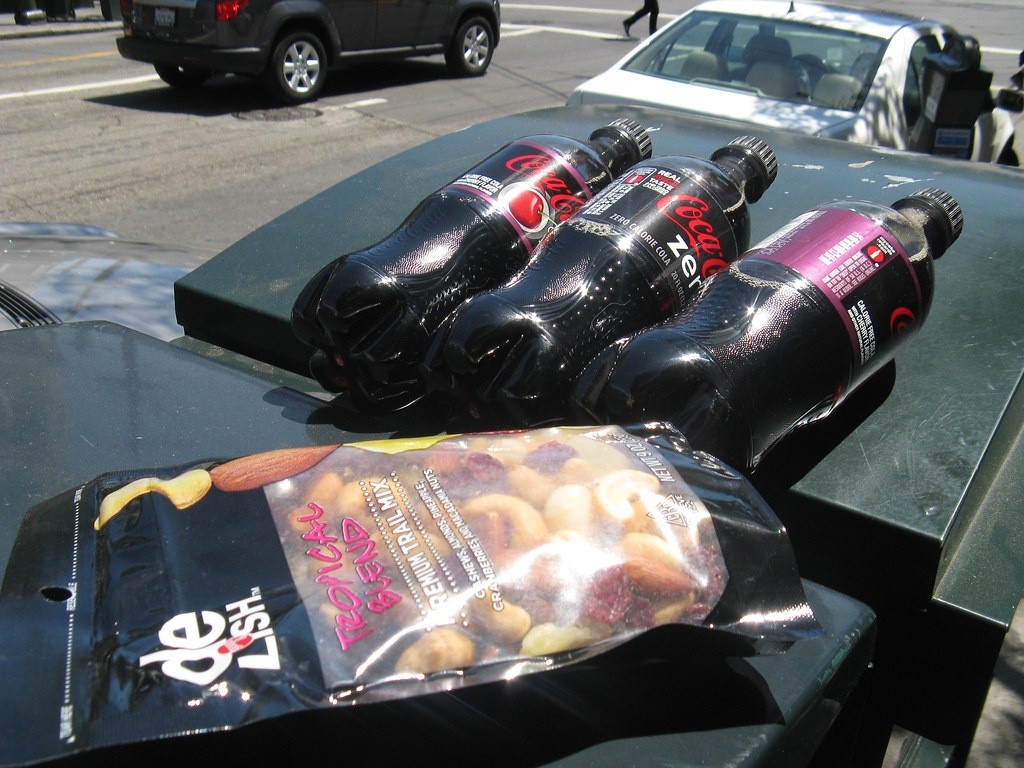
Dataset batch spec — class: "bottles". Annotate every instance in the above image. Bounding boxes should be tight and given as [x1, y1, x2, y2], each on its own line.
[417, 136, 777, 426]
[292, 116, 652, 415]
[567, 187, 964, 473]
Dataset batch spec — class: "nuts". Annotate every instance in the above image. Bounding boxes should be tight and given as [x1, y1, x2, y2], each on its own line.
[287, 427, 722, 676]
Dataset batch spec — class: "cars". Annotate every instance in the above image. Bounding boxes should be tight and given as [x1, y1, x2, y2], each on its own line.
[565, 0, 962, 161]
[117, 0, 503, 108]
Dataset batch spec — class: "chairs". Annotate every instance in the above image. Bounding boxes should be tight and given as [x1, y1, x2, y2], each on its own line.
[678, 36, 883, 111]
